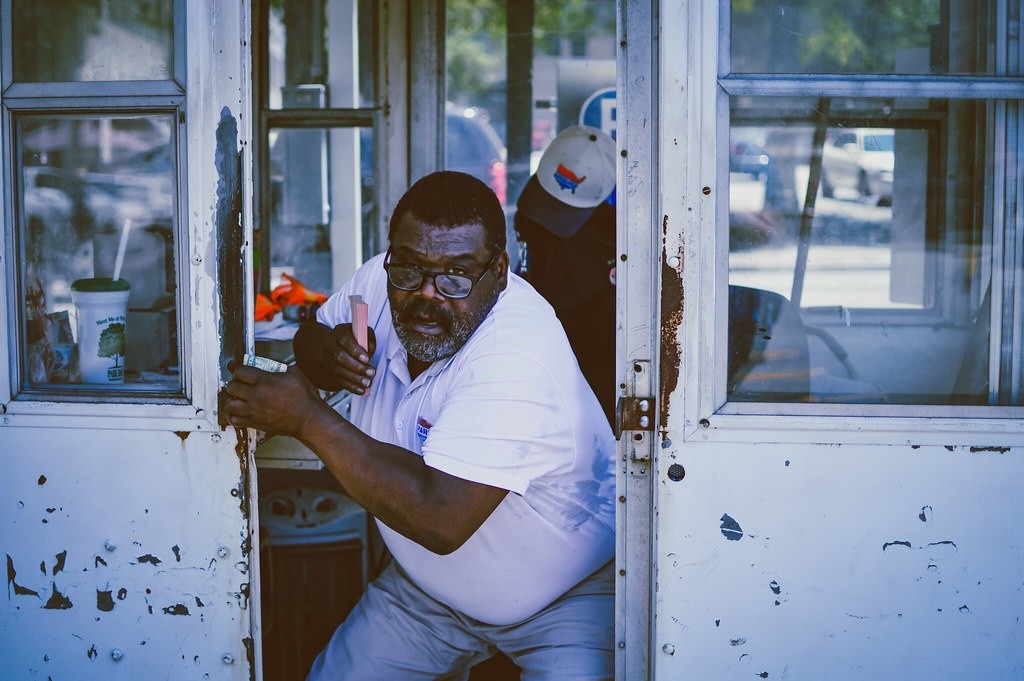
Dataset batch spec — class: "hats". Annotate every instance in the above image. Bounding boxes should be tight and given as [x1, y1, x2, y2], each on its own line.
[516, 124, 617, 239]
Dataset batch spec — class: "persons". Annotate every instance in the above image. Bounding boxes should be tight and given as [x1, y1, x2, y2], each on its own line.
[515, 126, 618, 434]
[224, 170, 615, 681]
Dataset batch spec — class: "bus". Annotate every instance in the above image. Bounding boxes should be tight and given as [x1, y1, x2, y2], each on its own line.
[0, 0, 1024, 681]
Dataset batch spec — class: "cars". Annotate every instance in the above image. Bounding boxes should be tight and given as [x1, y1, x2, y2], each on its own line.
[822, 128, 896, 207]
[762, 128, 818, 163]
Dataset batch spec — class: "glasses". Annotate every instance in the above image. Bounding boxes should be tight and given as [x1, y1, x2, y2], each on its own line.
[382, 244, 498, 299]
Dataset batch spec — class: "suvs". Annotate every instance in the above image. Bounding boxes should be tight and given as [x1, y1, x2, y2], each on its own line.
[263, 105, 510, 233]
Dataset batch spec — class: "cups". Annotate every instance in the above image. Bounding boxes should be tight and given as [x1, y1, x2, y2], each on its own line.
[70, 278, 130, 385]
